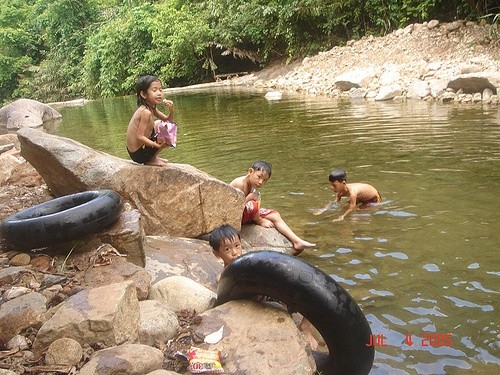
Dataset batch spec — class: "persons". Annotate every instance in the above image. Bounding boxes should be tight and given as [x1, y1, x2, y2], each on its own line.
[209, 223, 321, 352]
[228, 160, 317, 250]
[126, 75, 175, 168]
[313, 167, 381, 222]
